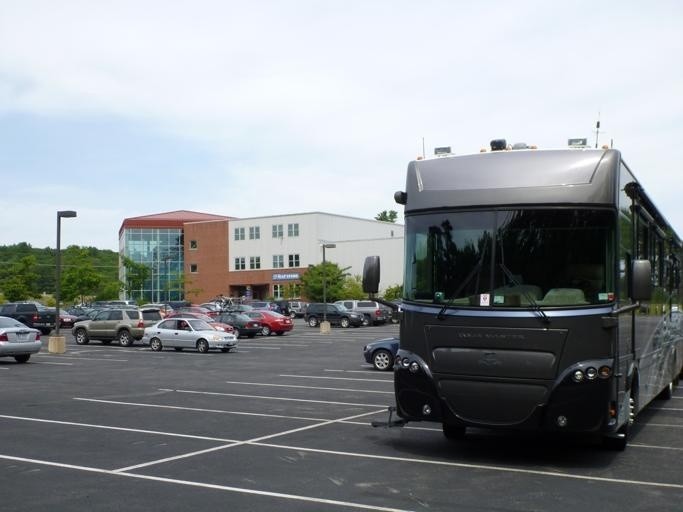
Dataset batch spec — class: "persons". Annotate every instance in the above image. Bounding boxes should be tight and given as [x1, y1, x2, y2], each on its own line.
[277, 298, 291, 316]
[159, 305, 170, 318]
[214, 293, 252, 310]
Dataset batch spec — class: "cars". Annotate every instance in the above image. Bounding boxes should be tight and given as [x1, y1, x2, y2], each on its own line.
[220, 296, 400, 339]
[162, 312, 237, 342]
[140, 317, 239, 353]
[0, 316, 43, 363]
[362, 334, 400, 370]
[64, 298, 220, 322]
[38, 306, 79, 327]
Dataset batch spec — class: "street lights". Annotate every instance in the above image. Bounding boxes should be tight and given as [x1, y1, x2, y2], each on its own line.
[321, 243, 336, 323]
[162, 255, 174, 316]
[53, 210, 76, 336]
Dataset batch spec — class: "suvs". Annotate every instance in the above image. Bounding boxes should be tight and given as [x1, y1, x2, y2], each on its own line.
[70, 307, 163, 346]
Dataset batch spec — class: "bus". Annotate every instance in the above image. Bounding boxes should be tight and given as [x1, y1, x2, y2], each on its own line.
[362, 115, 683, 453]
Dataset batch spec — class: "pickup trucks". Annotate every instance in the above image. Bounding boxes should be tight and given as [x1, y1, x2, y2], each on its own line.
[0, 303, 56, 336]
